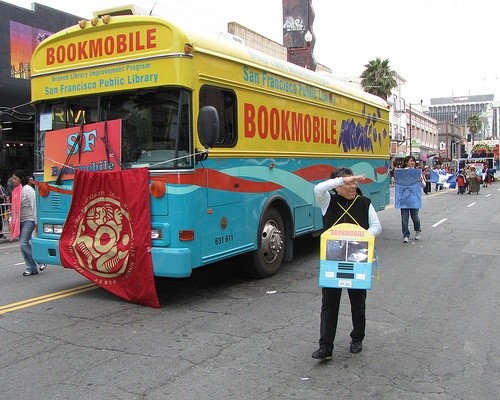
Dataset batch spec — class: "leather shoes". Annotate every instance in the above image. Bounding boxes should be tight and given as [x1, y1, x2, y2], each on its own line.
[312, 347, 334, 359]
[350, 340, 362, 353]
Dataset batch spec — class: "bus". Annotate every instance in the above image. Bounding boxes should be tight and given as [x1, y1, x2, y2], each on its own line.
[26, 2, 391, 278]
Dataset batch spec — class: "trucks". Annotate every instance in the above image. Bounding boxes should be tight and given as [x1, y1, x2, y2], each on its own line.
[452, 158, 495, 183]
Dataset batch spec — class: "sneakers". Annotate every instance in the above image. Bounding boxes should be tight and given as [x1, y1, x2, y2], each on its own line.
[415, 230, 422, 239]
[403, 237, 409, 242]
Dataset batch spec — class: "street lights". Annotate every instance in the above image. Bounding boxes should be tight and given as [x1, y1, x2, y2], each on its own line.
[408, 99, 424, 155]
[446, 104, 457, 159]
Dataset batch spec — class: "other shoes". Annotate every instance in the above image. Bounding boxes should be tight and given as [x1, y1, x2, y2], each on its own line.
[23, 270, 37, 275]
[39, 264, 46, 271]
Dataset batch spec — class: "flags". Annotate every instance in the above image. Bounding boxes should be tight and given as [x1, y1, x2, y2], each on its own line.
[58, 166, 160, 308]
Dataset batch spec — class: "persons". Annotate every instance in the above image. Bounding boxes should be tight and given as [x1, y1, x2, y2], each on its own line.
[27, 176, 33, 186]
[466, 167, 481, 194]
[11, 169, 47, 276]
[482, 164, 491, 187]
[460, 168, 468, 192]
[0, 177, 6, 239]
[400, 155, 423, 243]
[312, 168, 383, 360]
[388, 159, 458, 195]
[456, 170, 466, 194]
[7, 173, 15, 198]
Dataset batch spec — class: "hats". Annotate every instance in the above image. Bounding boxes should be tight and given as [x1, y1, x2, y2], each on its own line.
[470, 165, 475, 168]
